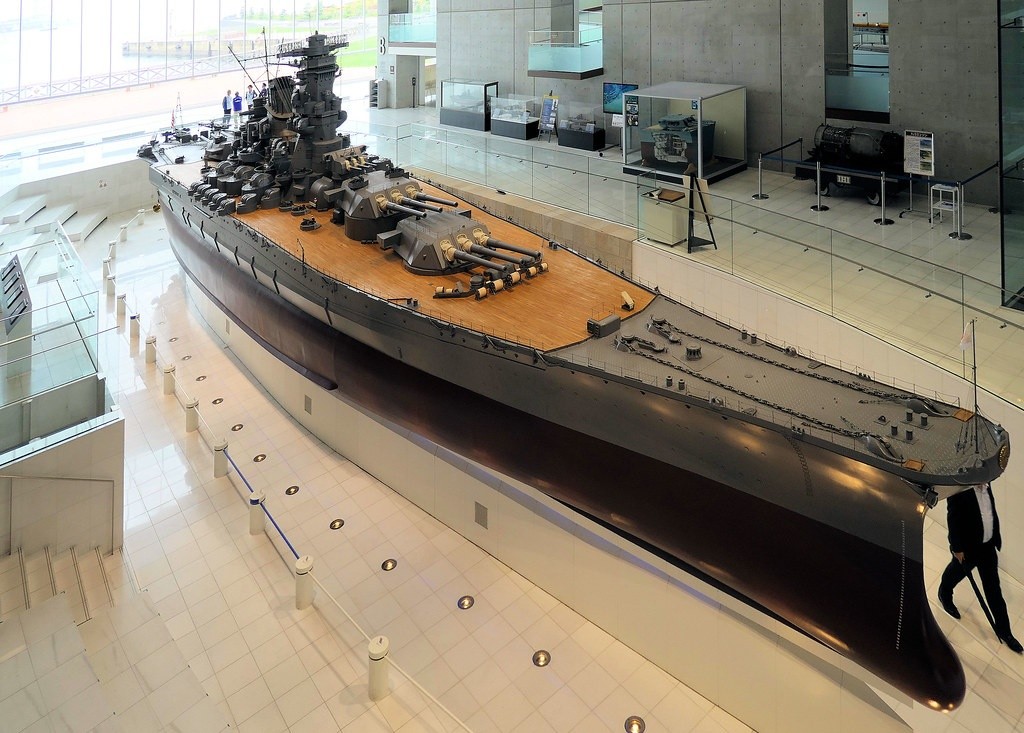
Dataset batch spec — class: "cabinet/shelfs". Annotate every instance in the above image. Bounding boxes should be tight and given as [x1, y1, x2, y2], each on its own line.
[930, 183, 965, 233]
[369, 79, 388, 109]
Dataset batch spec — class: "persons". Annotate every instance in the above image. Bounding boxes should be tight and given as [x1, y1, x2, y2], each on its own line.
[222, 83, 266, 126]
[938, 482, 1024, 653]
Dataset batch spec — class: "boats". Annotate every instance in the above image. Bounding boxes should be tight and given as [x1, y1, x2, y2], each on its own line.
[135, 24, 1011, 714]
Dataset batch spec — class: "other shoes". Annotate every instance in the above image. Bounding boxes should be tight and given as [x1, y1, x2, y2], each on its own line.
[994, 623, 1023, 653]
[938, 594, 961, 619]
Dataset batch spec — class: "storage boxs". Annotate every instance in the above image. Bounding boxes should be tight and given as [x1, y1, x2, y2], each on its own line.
[621, 81, 749, 186]
[490, 93, 542, 141]
[557, 101, 606, 151]
[440, 77, 499, 132]
